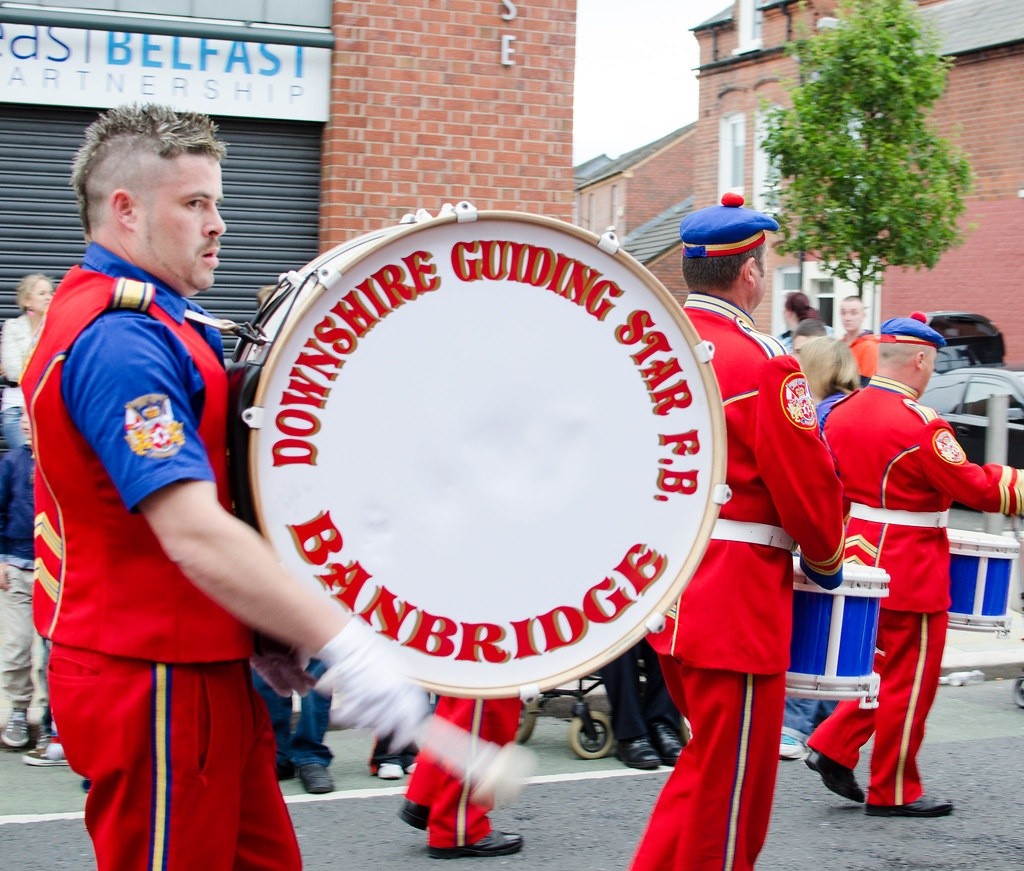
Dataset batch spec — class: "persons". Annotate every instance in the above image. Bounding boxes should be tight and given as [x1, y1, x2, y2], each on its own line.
[248, 657, 336, 792]
[836, 295, 879, 388]
[0, 402, 69, 766]
[793, 317, 828, 362]
[601, 637, 685, 768]
[395, 695, 524, 858]
[778, 334, 863, 762]
[19, 103, 421, 871]
[626, 192, 844, 871]
[369, 690, 430, 780]
[804, 311, 1024, 818]
[0, 273, 55, 450]
[775, 291, 819, 341]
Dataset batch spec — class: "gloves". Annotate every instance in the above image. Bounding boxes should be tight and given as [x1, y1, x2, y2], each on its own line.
[313, 616, 430, 758]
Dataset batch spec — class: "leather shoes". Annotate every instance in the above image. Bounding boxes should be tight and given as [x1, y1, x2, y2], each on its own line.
[299, 763, 334, 793]
[426, 830, 523, 860]
[805, 750, 865, 803]
[865, 798, 953, 817]
[617, 735, 664, 769]
[647, 722, 684, 765]
[276, 760, 296, 780]
[398, 799, 432, 831]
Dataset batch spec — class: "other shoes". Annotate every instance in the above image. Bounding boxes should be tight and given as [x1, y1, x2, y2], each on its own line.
[35, 725, 51, 750]
[24, 752, 70, 766]
[1, 709, 30, 747]
[378, 763, 404, 779]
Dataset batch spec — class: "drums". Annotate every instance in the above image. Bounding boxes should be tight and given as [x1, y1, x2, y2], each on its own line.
[787, 555, 895, 701]
[234, 205, 732, 699]
[945, 522, 1021, 638]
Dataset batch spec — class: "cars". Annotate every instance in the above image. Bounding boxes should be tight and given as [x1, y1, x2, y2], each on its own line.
[919, 363, 1024, 470]
[924, 312, 1007, 375]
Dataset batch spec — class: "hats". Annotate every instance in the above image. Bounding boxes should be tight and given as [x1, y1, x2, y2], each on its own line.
[879, 311, 948, 349]
[679, 191, 780, 258]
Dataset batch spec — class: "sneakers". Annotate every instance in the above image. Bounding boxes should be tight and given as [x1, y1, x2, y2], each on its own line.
[779, 734, 805, 760]
